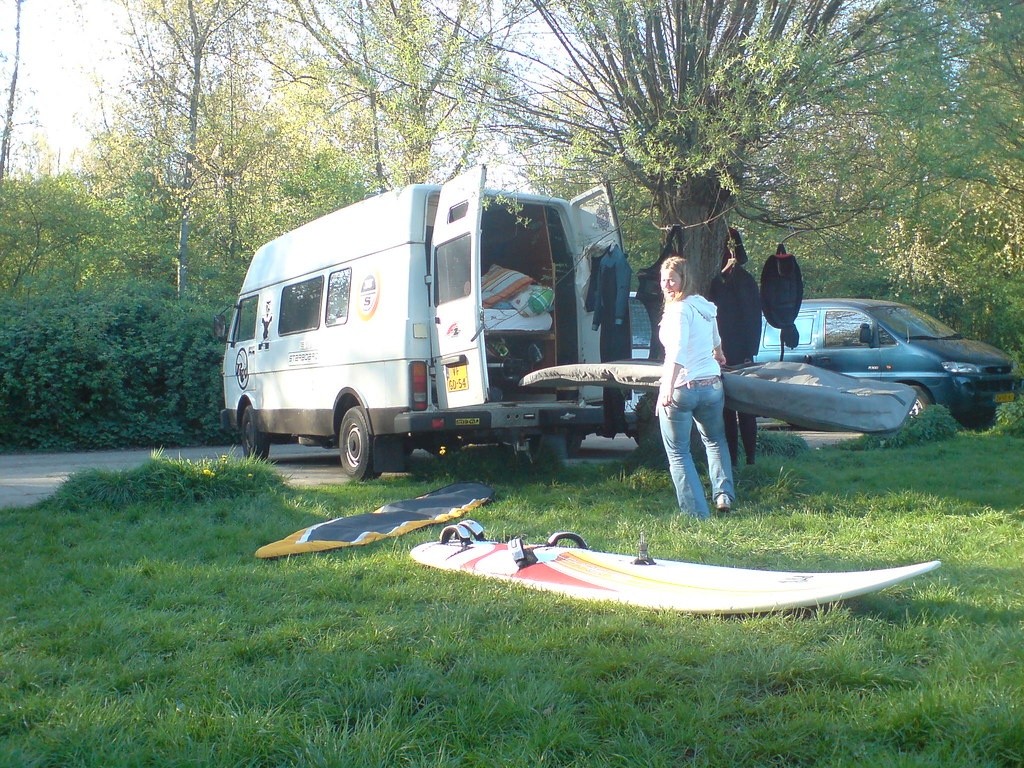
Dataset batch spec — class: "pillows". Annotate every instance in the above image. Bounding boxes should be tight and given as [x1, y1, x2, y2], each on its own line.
[481, 264, 534, 309]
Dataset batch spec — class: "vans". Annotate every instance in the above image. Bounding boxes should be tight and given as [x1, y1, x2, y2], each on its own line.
[215, 164, 633, 483]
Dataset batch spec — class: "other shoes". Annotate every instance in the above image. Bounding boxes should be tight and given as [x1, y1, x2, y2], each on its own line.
[716, 493, 731, 511]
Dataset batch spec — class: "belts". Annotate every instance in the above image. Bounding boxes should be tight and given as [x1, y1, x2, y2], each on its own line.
[683, 377, 720, 389]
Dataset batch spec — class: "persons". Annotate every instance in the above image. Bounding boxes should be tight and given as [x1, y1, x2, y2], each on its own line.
[655, 256, 736, 522]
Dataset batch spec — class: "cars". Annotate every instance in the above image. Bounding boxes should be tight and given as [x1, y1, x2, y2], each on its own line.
[753, 298, 1015, 429]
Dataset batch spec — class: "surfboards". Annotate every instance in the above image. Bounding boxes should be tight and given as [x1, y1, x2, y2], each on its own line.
[407, 519, 944, 614]
